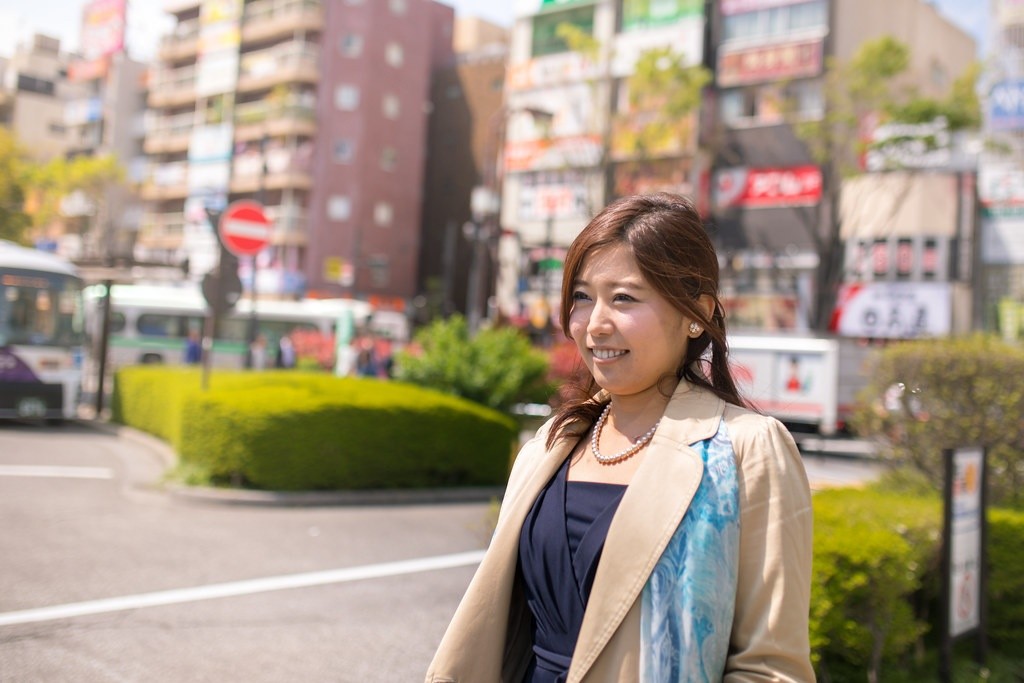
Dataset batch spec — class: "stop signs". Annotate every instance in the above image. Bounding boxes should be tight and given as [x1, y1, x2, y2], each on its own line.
[219, 201, 274, 256]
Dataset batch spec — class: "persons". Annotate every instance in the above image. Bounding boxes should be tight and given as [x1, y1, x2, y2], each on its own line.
[244, 333, 267, 371]
[185, 329, 204, 364]
[334, 338, 379, 380]
[423, 191, 819, 683]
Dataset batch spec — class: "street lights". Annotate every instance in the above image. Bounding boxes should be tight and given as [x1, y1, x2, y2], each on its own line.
[468, 106, 553, 337]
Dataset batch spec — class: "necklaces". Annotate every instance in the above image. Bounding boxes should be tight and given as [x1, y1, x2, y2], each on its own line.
[591, 401, 662, 465]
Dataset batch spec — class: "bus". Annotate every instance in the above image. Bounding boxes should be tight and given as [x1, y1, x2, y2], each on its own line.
[91, 285, 409, 378]
[0, 237, 88, 425]
